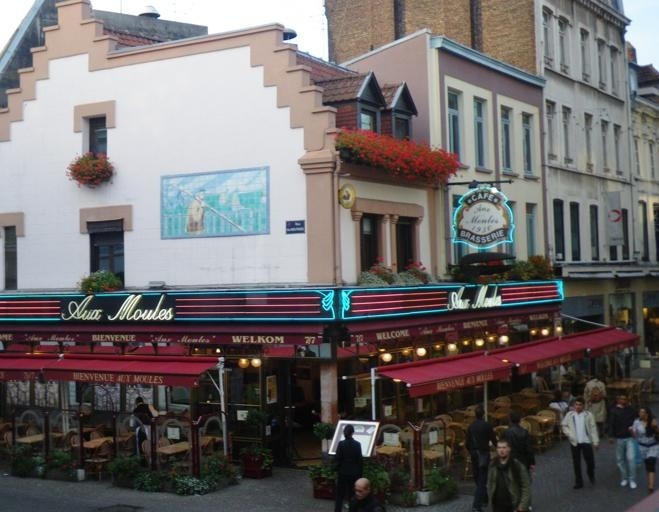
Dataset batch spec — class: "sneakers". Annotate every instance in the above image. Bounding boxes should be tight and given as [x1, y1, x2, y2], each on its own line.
[574, 481, 583, 488]
[588, 470, 594, 480]
[621, 480, 637, 489]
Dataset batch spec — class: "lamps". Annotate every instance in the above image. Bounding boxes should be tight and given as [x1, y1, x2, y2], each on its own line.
[380, 350, 394, 364]
[474, 337, 485, 348]
[530, 324, 564, 338]
[447, 343, 458, 352]
[238, 357, 261, 370]
[413, 348, 430, 358]
[500, 333, 511, 345]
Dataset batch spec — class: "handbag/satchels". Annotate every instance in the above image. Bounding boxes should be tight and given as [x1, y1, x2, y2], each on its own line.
[591, 387, 601, 403]
[491, 488, 513, 512]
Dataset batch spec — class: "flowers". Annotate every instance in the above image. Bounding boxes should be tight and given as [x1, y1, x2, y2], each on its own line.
[338, 127, 463, 185]
[66, 151, 116, 190]
[76, 266, 123, 297]
[359, 254, 400, 288]
[401, 255, 430, 284]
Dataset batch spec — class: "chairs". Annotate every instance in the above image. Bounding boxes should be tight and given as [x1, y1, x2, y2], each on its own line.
[307, 370, 654, 506]
[2, 421, 240, 497]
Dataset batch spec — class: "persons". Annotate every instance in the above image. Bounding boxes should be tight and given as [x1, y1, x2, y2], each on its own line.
[500, 412, 536, 511]
[464, 406, 497, 512]
[349, 478, 386, 512]
[129, 396, 159, 467]
[534, 362, 621, 438]
[628, 407, 659, 494]
[485, 438, 531, 512]
[333, 424, 362, 512]
[607, 392, 639, 489]
[185, 189, 206, 232]
[560, 397, 601, 490]
